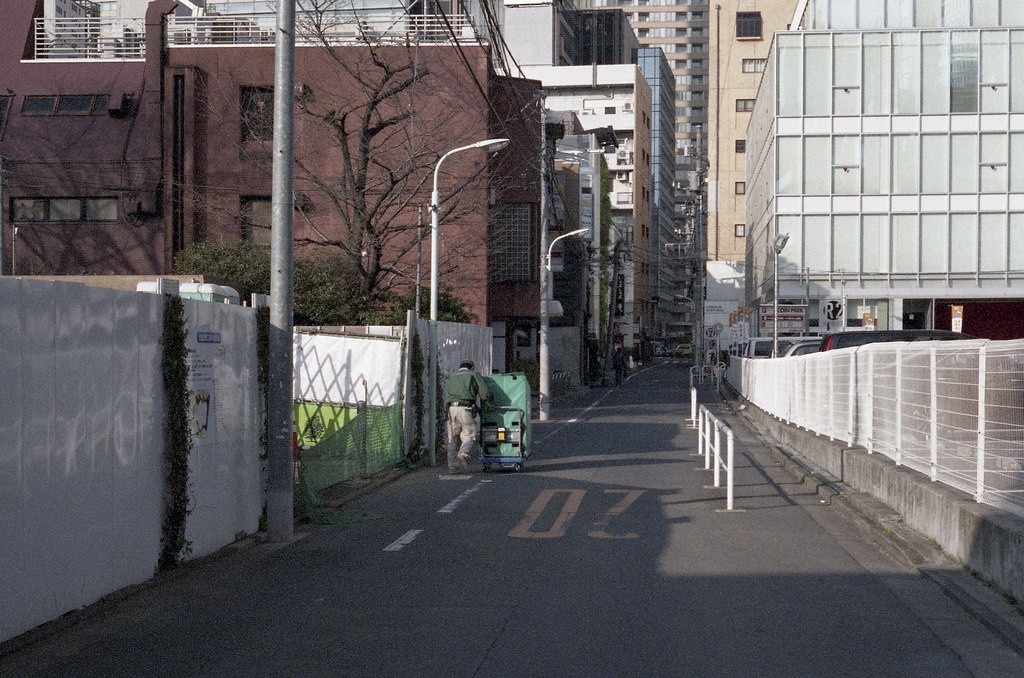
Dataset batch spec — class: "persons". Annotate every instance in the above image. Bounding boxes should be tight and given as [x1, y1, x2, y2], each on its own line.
[613, 347, 627, 387]
[447, 360, 493, 474]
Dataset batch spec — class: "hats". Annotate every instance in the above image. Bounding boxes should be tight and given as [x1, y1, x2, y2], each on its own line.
[461, 359, 475, 365]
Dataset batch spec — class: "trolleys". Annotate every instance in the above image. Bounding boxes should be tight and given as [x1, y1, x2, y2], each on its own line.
[479, 372, 531, 472]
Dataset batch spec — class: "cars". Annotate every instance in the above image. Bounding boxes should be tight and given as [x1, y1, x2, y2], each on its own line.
[672, 344, 693, 357]
[779, 340, 822, 357]
[819, 330, 980, 352]
[652, 341, 666, 357]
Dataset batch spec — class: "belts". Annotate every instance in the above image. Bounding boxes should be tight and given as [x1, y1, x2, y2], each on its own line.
[451, 401, 468, 407]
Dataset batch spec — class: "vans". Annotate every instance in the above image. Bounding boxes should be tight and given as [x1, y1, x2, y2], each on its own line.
[748, 336, 823, 359]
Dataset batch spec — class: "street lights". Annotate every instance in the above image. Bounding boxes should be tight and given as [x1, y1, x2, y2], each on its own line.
[772, 233, 790, 358]
[428, 138, 510, 467]
[675, 293, 697, 365]
[540, 227, 592, 420]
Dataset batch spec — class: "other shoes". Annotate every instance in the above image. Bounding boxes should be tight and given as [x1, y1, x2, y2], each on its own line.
[456, 454, 470, 466]
[451, 468, 460, 474]
